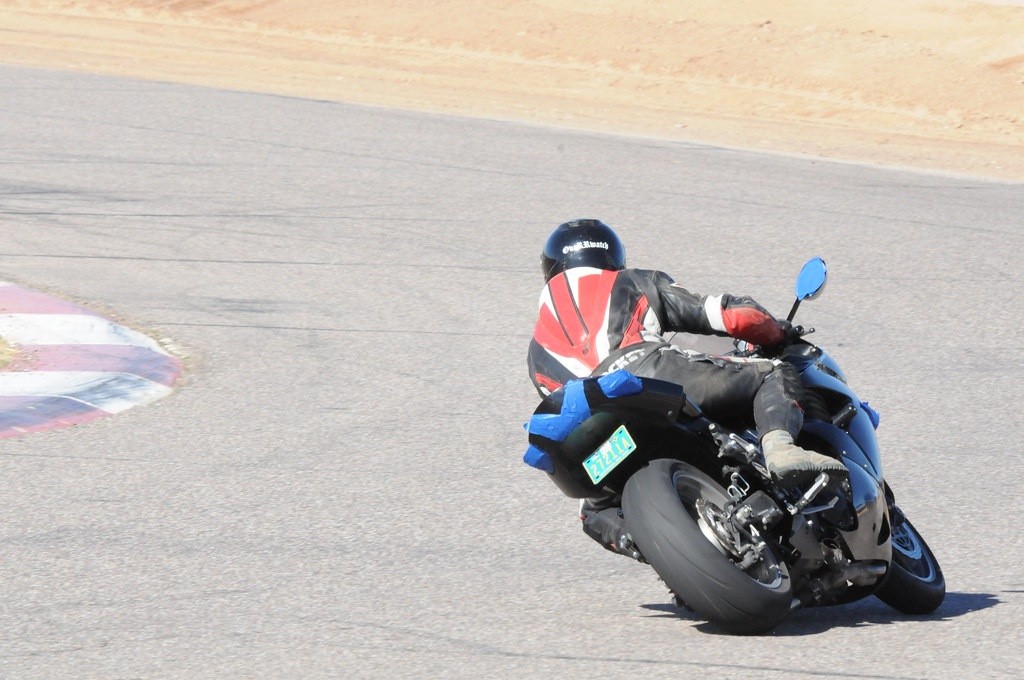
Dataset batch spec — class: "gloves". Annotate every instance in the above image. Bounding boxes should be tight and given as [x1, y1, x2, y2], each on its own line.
[754, 319, 792, 358]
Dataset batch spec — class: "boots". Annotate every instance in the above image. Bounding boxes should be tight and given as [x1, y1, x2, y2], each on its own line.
[761, 429, 850, 484]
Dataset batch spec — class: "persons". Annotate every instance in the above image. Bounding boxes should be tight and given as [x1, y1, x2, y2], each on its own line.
[527, 219, 849, 494]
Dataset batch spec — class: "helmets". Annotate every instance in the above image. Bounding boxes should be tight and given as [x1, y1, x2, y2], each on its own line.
[541, 218, 626, 284]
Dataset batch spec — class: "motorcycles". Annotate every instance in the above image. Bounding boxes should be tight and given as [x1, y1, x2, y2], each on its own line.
[522, 256, 946, 635]
